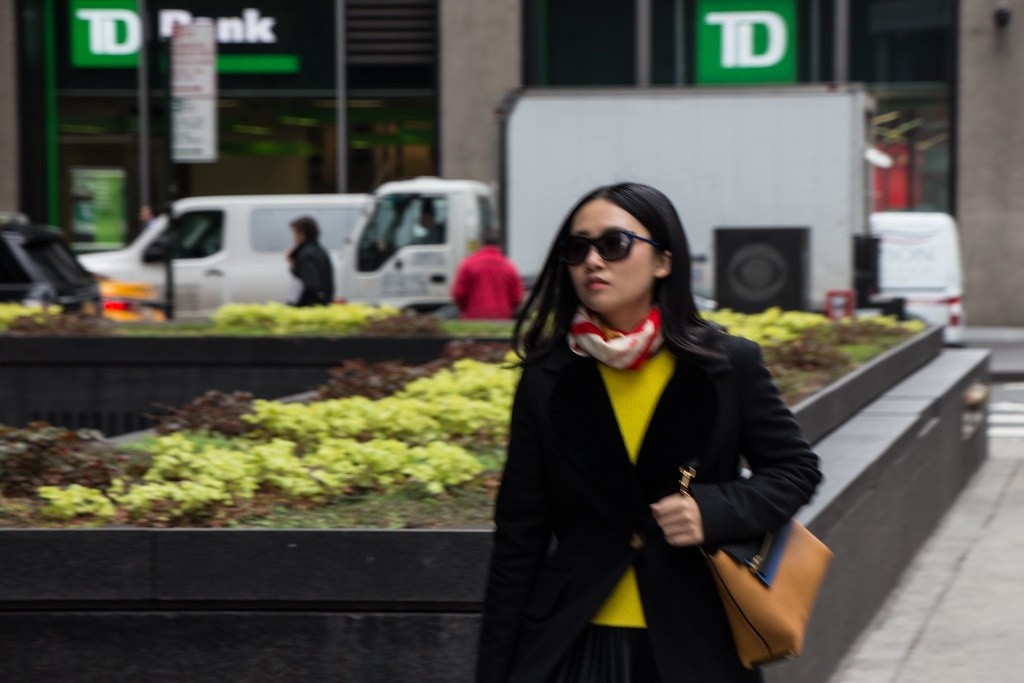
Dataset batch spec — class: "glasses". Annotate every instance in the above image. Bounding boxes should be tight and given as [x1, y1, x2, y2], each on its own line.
[556, 230, 666, 265]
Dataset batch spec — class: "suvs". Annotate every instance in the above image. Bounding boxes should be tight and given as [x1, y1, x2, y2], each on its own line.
[0, 221, 106, 318]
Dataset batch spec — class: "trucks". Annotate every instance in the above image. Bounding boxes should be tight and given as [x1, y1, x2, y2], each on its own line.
[344, 82, 880, 320]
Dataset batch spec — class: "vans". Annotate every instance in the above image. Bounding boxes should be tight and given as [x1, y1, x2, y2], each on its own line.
[870, 210, 964, 343]
[76, 194, 373, 317]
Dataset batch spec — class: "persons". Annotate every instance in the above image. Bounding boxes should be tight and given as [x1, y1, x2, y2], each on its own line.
[287, 217, 334, 308]
[475, 183, 824, 683]
[451, 232, 523, 321]
[142, 206, 166, 252]
[413, 202, 443, 244]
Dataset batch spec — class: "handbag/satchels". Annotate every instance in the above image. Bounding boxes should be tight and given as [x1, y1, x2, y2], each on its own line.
[706, 518, 836, 670]
